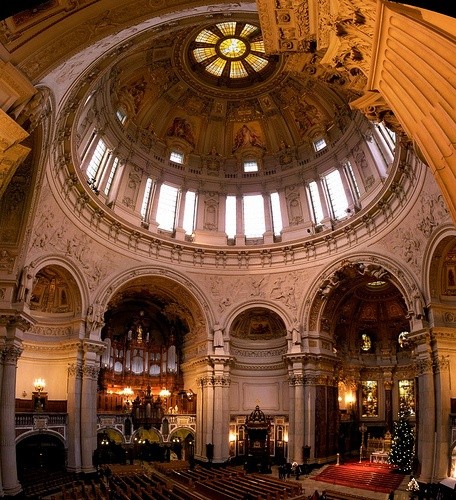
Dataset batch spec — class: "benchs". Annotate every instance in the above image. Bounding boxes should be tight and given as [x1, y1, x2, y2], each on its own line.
[17, 465, 306, 500]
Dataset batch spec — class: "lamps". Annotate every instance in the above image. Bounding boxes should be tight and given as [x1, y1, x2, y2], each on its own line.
[32, 379, 46, 393]
